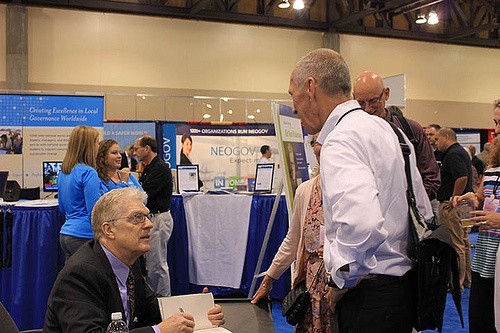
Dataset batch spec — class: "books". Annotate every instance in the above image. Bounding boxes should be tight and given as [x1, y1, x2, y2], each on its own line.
[157, 292, 232, 333]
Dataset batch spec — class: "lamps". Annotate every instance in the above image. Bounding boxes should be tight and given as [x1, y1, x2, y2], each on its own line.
[414, 6, 441, 26]
[277, 0, 307, 11]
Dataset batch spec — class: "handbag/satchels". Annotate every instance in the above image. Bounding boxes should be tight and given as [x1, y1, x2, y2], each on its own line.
[282, 280, 311, 325]
[410, 234, 466, 333]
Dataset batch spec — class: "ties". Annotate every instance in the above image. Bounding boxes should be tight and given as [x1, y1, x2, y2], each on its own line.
[125, 269, 141, 330]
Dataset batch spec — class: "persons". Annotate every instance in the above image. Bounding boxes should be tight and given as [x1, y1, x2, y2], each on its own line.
[288, 49, 433, 333]
[451, 101, 500, 333]
[260, 145, 272, 162]
[45, 163, 62, 173]
[463, 147, 478, 192]
[467, 144, 483, 174]
[478, 142, 491, 164]
[180, 133, 193, 165]
[433, 128, 474, 292]
[424, 124, 441, 159]
[250, 133, 332, 333]
[96, 138, 148, 205]
[133, 135, 174, 297]
[39, 186, 226, 333]
[0, 129, 22, 155]
[353, 71, 442, 197]
[57, 126, 108, 263]
[119, 144, 138, 171]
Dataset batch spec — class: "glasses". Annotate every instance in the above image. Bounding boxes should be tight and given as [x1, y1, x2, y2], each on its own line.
[133, 146, 144, 151]
[353, 88, 385, 107]
[103, 212, 155, 225]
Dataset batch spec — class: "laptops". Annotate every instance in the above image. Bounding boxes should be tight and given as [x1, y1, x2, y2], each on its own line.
[237, 163, 275, 194]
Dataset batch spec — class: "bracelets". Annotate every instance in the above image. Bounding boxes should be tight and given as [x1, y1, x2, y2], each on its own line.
[474, 194, 480, 201]
[326, 276, 338, 288]
[261, 282, 273, 291]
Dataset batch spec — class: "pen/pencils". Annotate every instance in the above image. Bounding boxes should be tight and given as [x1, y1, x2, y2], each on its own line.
[179, 308, 184, 313]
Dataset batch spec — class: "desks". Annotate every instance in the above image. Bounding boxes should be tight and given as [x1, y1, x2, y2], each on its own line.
[168, 193, 292, 301]
[215, 303, 276, 333]
[0, 199, 65, 329]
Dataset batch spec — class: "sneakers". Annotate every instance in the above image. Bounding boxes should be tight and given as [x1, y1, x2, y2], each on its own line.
[445, 278, 472, 294]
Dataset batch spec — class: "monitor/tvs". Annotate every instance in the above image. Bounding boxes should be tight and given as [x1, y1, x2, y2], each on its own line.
[177, 164, 200, 194]
[455, 131, 484, 155]
[42, 161, 64, 192]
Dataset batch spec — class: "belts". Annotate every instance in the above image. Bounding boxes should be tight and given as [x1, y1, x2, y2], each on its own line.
[149, 208, 169, 214]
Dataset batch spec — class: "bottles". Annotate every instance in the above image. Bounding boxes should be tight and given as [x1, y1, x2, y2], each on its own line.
[106, 312, 129, 333]
[457, 198, 476, 228]
